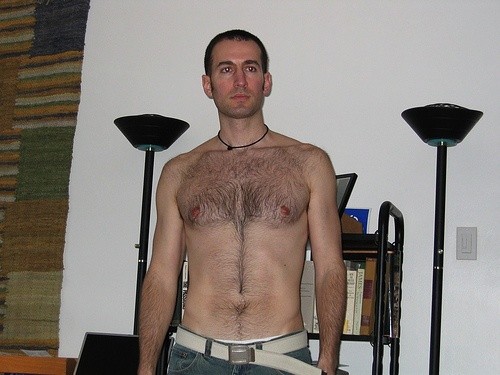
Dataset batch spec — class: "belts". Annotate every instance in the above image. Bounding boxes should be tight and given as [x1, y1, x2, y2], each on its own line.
[175, 328, 322, 375]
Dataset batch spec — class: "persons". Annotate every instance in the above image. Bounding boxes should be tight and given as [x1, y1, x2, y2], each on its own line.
[138, 29, 345, 375]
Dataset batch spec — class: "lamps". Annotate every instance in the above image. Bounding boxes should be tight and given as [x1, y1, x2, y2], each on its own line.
[113, 114, 191, 336]
[402, 103, 484, 374]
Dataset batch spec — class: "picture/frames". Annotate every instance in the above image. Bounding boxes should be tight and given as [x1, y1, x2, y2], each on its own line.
[336, 173, 358, 218]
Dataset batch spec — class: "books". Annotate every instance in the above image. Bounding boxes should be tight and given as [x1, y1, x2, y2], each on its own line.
[299, 260, 320, 334]
[343, 257, 378, 335]
[181, 261, 188, 321]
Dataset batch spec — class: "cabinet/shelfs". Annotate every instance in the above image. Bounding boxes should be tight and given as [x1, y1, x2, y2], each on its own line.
[154, 201, 405, 375]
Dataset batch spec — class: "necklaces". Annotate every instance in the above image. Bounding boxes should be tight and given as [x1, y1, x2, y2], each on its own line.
[217, 123, 269, 151]
